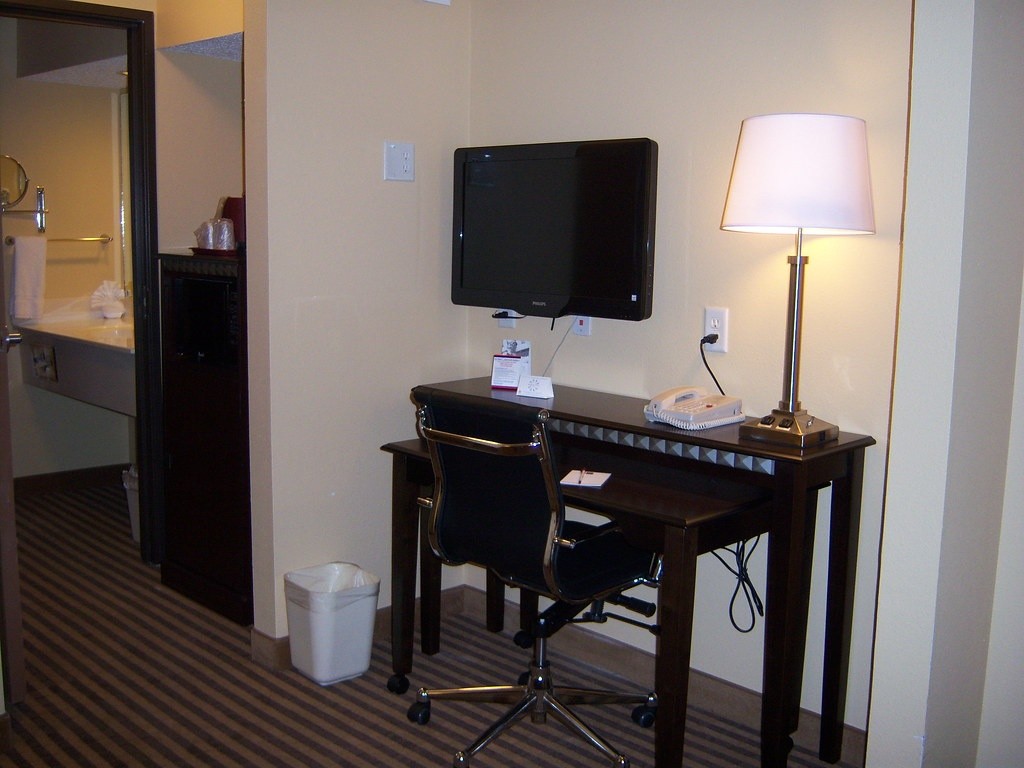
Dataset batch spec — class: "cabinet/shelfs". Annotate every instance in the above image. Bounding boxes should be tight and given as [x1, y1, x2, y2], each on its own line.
[156, 252, 255, 631]
[412, 374, 879, 767]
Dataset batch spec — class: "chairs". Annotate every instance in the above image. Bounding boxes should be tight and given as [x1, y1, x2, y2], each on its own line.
[407, 402, 657, 768]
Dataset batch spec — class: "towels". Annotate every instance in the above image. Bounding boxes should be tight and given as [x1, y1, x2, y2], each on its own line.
[7, 234, 48, 323]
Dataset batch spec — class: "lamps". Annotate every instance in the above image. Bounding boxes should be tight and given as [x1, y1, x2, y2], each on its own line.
[720, 112, 877, 446]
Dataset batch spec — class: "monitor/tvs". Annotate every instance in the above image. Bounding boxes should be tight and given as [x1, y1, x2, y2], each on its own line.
[450, 136, 659, 322]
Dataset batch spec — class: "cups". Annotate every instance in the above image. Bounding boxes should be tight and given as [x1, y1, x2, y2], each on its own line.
[198, 220, 235, 250]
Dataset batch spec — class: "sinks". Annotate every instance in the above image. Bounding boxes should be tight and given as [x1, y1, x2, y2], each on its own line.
[72, 320, 135, 347]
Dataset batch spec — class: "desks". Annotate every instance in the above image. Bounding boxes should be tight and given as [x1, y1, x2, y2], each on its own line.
[383, 439, 831, 767]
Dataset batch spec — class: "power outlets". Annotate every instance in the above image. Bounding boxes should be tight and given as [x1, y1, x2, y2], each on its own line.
[383, 139, 416, 183]
[497, 308, 515, 329]
[572, 315, 592, 337]
[703, 306, 729, 353]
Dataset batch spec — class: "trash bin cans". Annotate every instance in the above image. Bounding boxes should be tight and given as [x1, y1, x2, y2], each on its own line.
[283, 560, 379, 687]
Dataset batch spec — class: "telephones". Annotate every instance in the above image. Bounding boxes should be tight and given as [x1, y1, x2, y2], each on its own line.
[643, 386, 745, 431]
